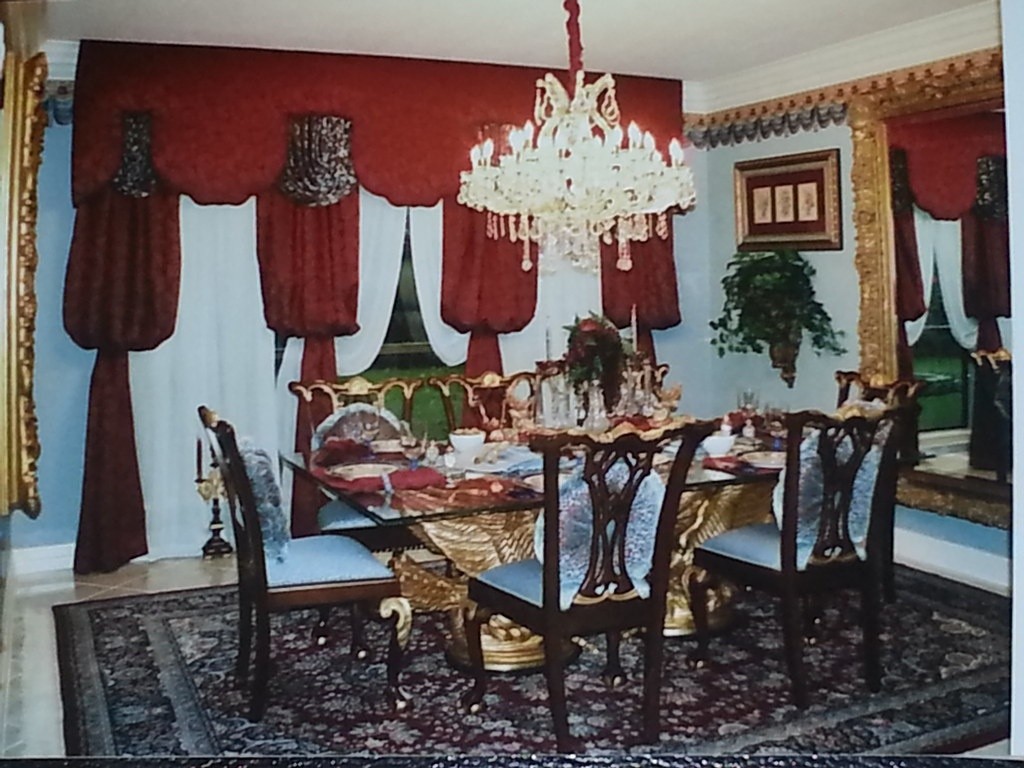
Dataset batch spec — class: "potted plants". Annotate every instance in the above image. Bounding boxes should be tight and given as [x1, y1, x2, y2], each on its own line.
[708, 249, 847, 389]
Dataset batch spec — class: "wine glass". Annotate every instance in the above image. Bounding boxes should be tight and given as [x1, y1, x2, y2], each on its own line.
[765, 402, 790, 450]
[400, 420, 427, 471]
[343, 406, 380, 461]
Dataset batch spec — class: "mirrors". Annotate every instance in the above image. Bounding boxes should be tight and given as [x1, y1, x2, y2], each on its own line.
[849, 64, 1013, 528]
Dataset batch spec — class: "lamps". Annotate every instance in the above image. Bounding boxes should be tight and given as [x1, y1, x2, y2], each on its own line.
[458, 0, 697, 273]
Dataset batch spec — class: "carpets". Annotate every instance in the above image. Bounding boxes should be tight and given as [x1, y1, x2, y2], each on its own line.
[50, 559, 1010, 758]
[282, 451, 936, 713]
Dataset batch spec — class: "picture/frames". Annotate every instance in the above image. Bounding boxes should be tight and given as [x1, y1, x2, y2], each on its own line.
[732, 148, 843, 253]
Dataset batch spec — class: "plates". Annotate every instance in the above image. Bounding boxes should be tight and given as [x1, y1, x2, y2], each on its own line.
[524, 474, 572, 493]
[327, 464, 397, 477]
[738, 452, 787, 468]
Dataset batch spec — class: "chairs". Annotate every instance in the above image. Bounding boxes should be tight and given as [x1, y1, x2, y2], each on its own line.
[431, 372, 549, 437]
[286, 377, 425, 451]
[196, 405, 402, 724]
[834, 370, 926, 603]
[467, 418, 724, 741]
[688, 402, 921, 709]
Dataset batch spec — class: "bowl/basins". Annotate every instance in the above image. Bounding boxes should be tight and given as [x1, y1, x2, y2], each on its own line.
[702, 434, 737, 458]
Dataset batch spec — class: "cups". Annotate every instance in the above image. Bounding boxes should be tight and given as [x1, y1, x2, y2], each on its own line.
[449, 431, 486, 453]
[737, 386, 760, 418]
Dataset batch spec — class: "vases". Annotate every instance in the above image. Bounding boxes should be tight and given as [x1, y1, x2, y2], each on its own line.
[584, 382, 611, 434]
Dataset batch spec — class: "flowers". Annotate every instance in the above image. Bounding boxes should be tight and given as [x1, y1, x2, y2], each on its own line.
[564, 310, 623, 384]
